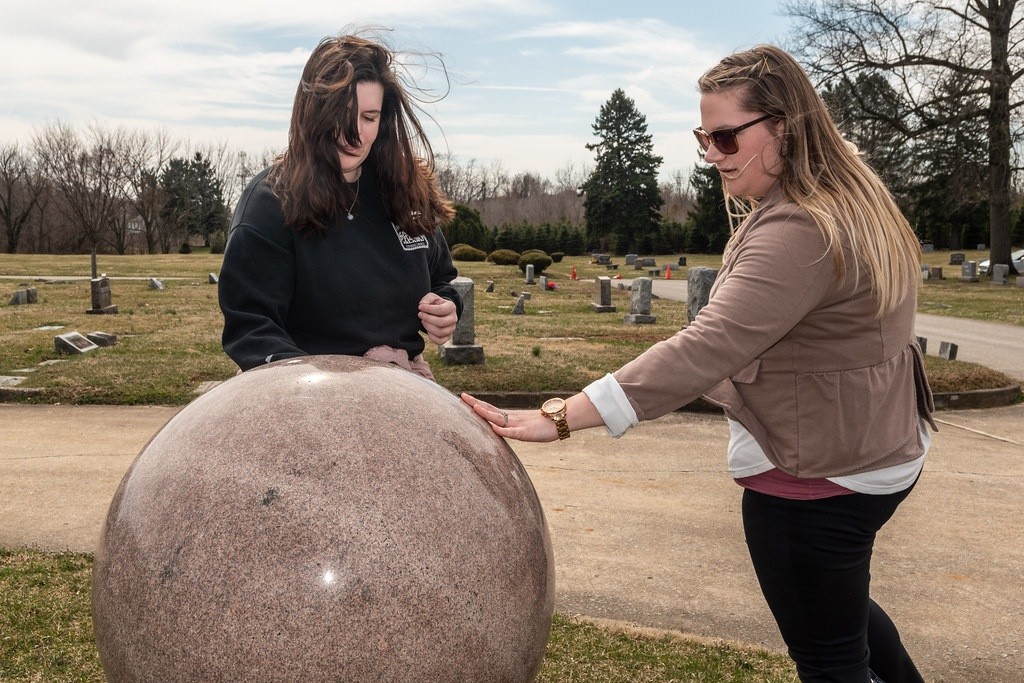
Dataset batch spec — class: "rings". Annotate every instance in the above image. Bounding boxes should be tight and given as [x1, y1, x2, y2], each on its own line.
[502, 412, 509, 428]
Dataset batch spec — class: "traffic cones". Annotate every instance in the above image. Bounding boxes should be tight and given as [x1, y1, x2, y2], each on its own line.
[665, 262, 673, 280]
[570, 265, 577, 280]
[614, 274, 622, 279]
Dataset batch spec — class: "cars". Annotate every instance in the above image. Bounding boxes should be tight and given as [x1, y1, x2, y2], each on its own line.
[977, 249, 1024, 275]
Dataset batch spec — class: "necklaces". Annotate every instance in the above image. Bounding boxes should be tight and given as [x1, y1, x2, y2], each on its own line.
[345, 170, 359, 220]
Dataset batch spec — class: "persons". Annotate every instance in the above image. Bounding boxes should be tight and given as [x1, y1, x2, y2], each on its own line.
[216, 34, 463, 377]
[461, 46, 940, 683]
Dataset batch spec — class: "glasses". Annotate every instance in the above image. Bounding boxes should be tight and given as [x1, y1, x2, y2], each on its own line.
[692, 113, 779, 154]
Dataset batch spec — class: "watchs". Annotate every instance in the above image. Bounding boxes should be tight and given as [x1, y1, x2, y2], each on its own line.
[540, 397, 571, 440]
